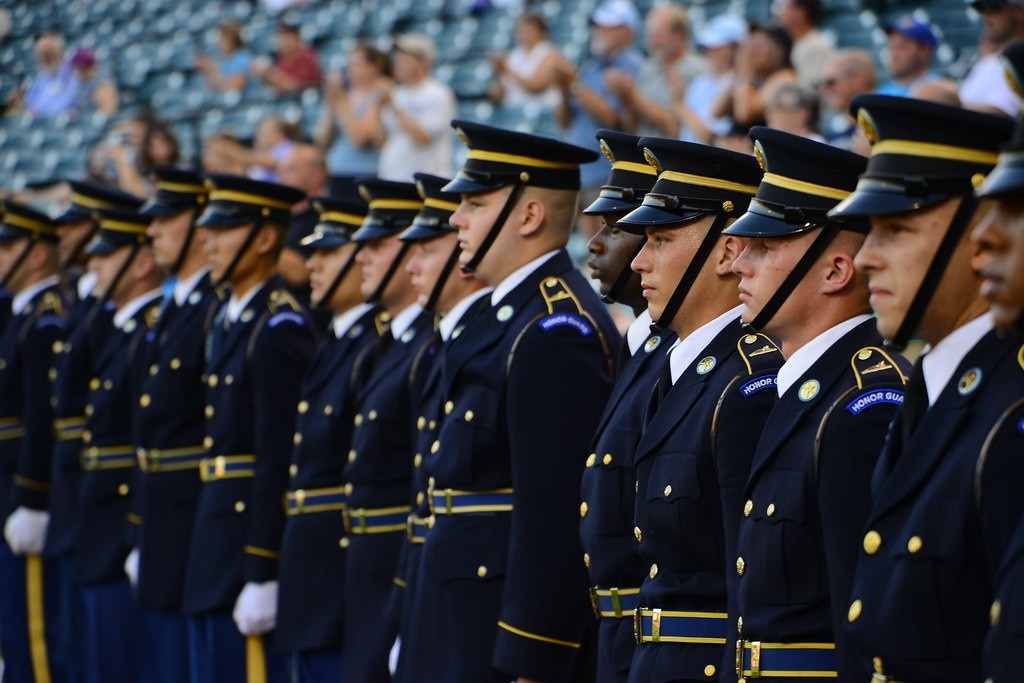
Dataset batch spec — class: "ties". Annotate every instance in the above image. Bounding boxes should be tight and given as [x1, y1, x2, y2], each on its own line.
[7, 297, 929, 470]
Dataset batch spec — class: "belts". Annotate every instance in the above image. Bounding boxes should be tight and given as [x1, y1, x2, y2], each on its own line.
[0, 416, 841, 678]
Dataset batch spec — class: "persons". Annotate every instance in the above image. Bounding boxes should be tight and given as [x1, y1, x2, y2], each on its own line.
[318, 33, 454, 183]
[486, 0, 1024, 131]
[581, 129, 786, 683]
[4, 31, 118, 122]
[419, 118, 629, 683]
[274, 171, 515, 682]
[0, 163, 308, 683]
[827, 92, 1024, 683]
[94, 111, 328, 201]
[204, 21, 323, 91]
[721, 123, 915, 683]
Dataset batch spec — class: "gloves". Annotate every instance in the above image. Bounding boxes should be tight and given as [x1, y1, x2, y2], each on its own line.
[3, 506, 50, 556]
[126, 548, 144, 591]
[234, 583, 282, 639]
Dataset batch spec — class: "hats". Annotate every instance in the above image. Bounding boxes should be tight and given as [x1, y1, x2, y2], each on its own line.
[882, 18, 936, 51]
[2, 93, 1024, 242]
[590, 0, 643, 36]
[391, 36, 439, 65]
[695, 14, 748, 58]
